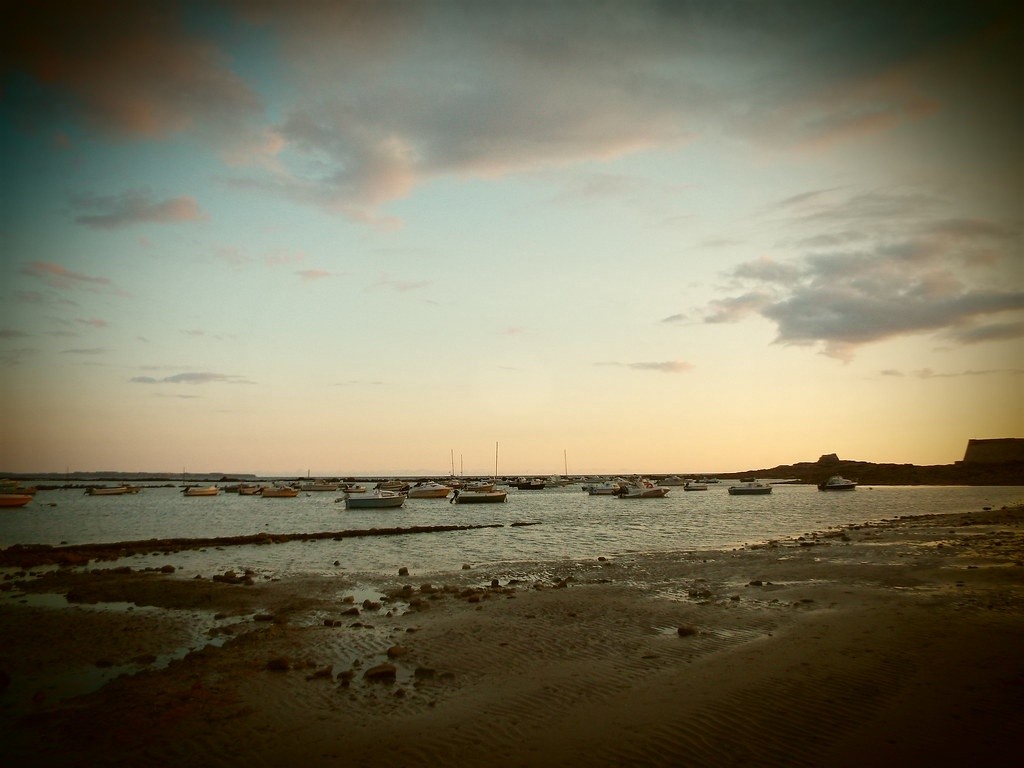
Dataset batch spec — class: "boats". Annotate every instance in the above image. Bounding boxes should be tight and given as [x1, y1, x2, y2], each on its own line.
[1, 477, 37, 508]
[295, 479, 367, 494]
[447, 442, 571, 490]
[85, 485, 141, 495]
[407, 480, 451, 498]
[571, 474, 671, 498]
[344, 494, 407, 509]
[374, 481, 410, 493]
[818, 473, 859, 490]
[655, 474, 721, 491]
[223, 480, 301, 497]
[453, 492, 507, 503]
[181, 485, 221, 495]
[727, 476, 774, 495]
